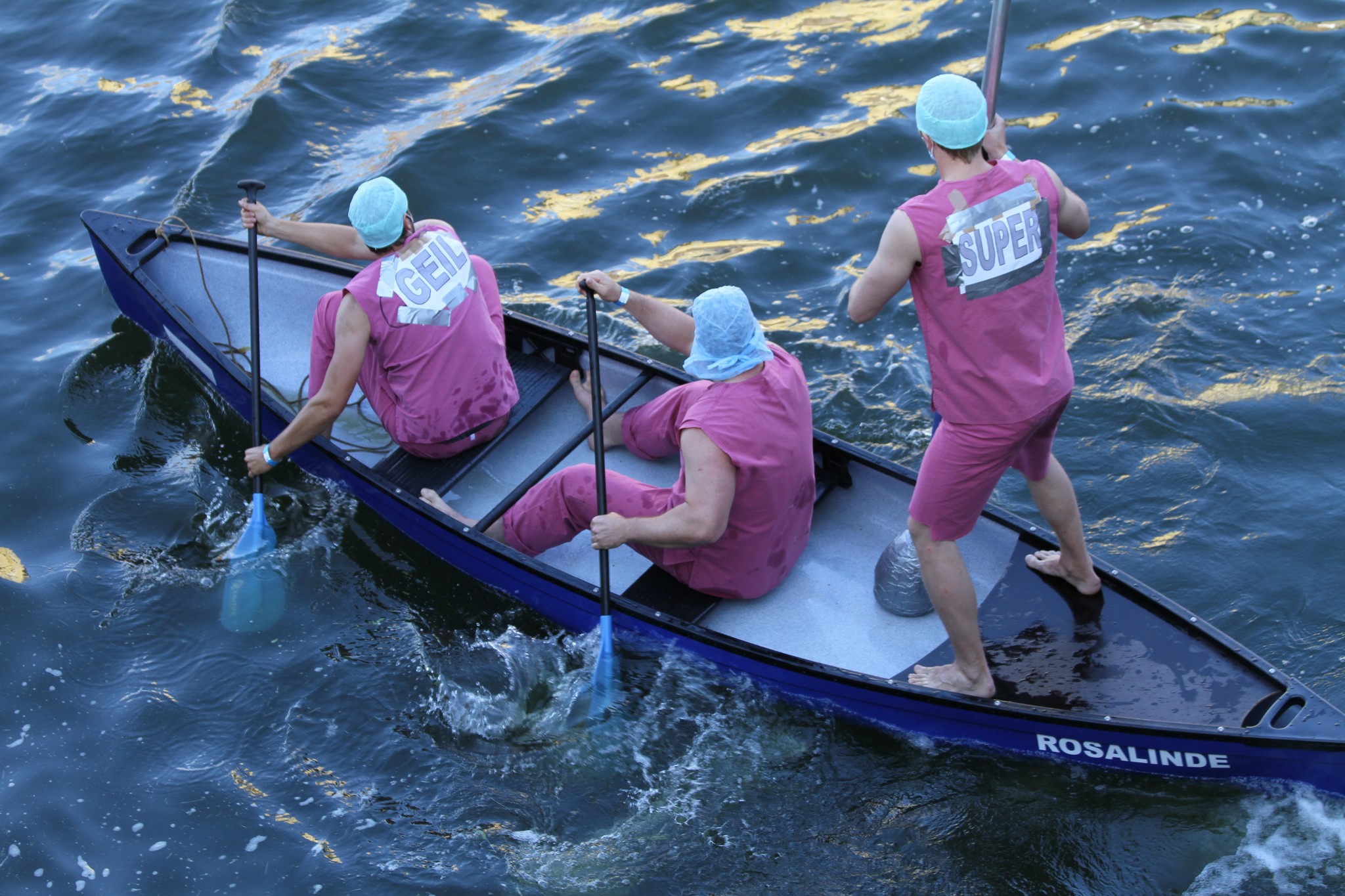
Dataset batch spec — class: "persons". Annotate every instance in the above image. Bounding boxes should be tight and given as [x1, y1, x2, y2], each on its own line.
[204, 174, 521, 481]
[418, 269, 816, 598]
[848, 74, 1101, 698]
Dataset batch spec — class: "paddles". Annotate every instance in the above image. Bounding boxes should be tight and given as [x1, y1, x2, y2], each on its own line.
[578, 279, 624, 717]
[219, 179, 286, 635]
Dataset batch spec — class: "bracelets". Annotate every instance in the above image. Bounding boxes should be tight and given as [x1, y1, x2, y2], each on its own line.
[613, 286, 630, 307]
[999, 150, 1016, 162]
[262, 443, 283, 467]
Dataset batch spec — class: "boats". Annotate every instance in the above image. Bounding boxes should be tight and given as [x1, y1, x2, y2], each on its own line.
[78, 207, 1344, 796]
